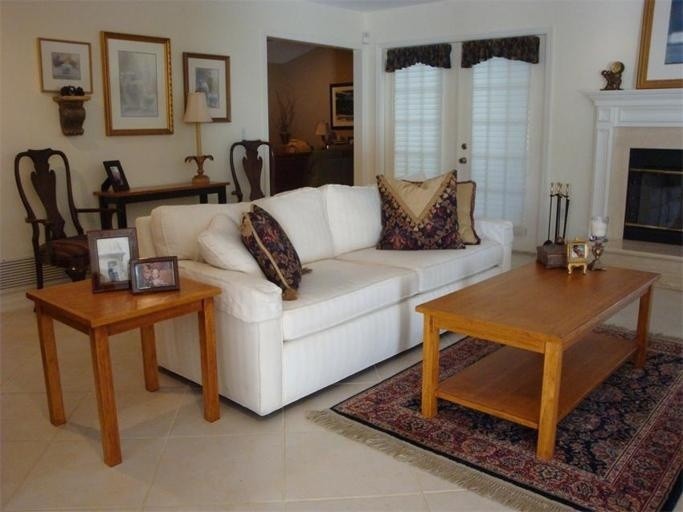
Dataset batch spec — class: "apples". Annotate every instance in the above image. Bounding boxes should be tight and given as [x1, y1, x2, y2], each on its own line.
[591, 218, 607, 237]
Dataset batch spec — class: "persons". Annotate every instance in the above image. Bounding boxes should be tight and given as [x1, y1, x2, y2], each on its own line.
[108, 261, 119, 281]
[572, 245, 586, 258]
[143, 265, 168, 287]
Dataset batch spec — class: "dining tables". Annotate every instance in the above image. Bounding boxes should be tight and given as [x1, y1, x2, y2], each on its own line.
[277, 87, 295, 144]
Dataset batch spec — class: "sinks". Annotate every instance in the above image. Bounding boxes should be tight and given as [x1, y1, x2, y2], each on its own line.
[135, 179, 513, 418]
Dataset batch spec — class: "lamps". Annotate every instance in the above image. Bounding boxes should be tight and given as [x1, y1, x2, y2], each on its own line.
[229, 139, 282, 203]
[14, 147, 116, 312]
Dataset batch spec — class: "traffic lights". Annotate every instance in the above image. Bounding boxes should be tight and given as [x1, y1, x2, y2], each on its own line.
[375, 169, 466, 249]
[240, 203, 312, 300]
[253, 186, 336, 266]
[320, 184, 383, 255]
[456, 180, 481, 246]
[197, 212, 268, 280]
[150, 203, 239, 260]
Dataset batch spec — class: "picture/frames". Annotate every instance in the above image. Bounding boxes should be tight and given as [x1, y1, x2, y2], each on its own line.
[93, 181, 230, 230]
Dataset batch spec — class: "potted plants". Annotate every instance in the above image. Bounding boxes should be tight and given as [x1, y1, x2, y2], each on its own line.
[635, 0, 683, 89]
[101, 160, 130, 192]
[130, 256, 180, 295]
[566, 240, 590, 264]
[38, 37, 93, 94]
[87, 227, 139, 293]
[183, 51, 232, 123]
[329, 82, 353, 129]
[100, 30, 175, 136]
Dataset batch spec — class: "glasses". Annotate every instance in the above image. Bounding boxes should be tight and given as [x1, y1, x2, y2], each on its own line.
[183, 93, 214, 183]
[315, 121, 330, 151]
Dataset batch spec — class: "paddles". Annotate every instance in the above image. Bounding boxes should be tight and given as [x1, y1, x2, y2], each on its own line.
[305, 320, 683, 512]
